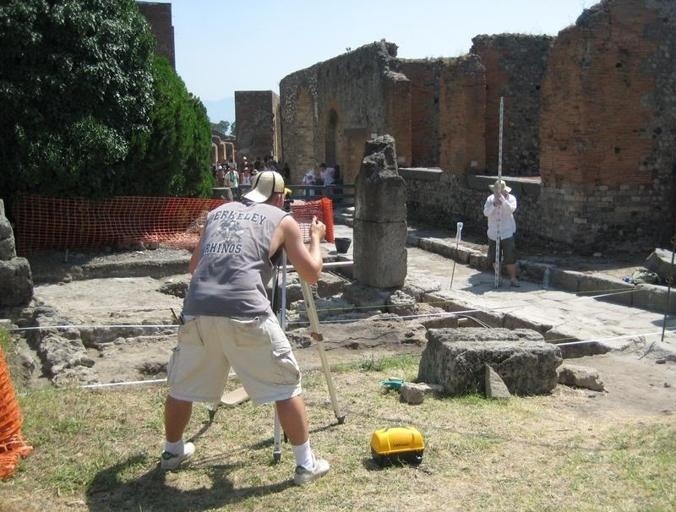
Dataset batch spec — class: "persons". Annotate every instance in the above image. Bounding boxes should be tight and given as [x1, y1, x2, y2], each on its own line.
[211, 153, 343, 209]
[482, 178, 522, 287]
[159, 168, 332, 488]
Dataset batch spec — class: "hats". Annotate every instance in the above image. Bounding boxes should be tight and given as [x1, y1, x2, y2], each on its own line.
[241, 171, 285, 203]
[488, 180, 512, 196]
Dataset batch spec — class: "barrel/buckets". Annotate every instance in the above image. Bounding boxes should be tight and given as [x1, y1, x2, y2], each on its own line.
[334, 238, 351, 252]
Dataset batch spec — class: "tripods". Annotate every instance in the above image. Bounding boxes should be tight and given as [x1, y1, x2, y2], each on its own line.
[208, 211, 346, 464]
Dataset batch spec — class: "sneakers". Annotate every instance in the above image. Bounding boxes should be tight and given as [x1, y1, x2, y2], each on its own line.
[293, 452, 331, 486]
[510, 279, 520, 287]
[160, 442, 196, 471]
